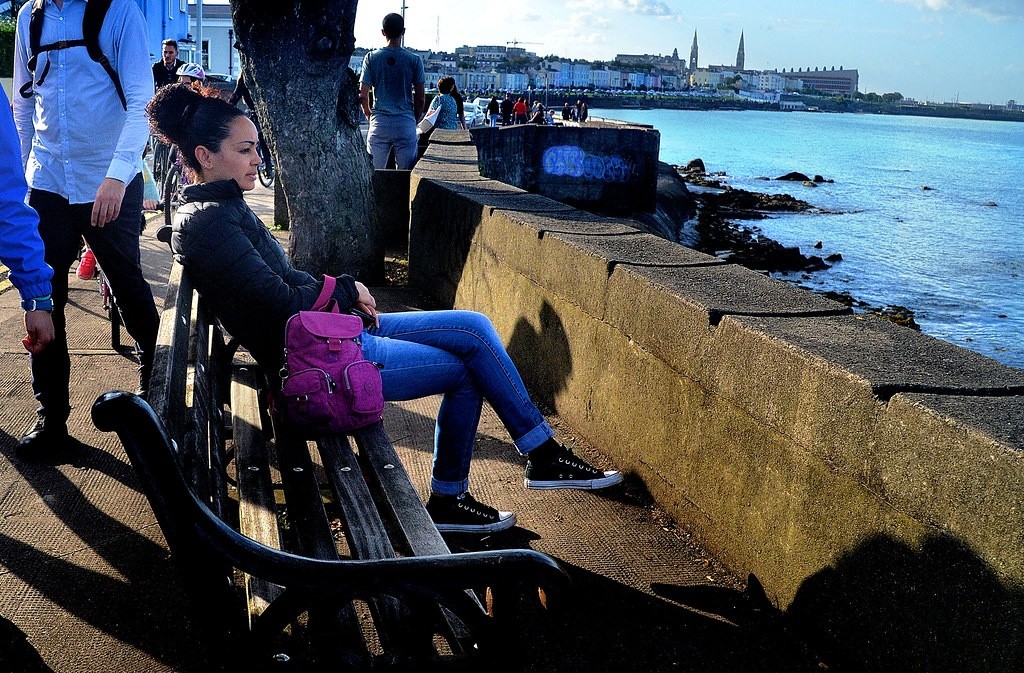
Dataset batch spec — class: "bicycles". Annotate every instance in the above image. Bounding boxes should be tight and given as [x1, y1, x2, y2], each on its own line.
[152, 134, 182, 222]
[95, 272, 121, 350]
[257, 140, 275, 188]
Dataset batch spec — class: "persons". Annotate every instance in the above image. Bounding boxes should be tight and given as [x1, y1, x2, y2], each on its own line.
[143, 84, 626, 530]
[151, 12, 590, 201]
[16, 0, 162, 456]
[0, 80, 57, 354]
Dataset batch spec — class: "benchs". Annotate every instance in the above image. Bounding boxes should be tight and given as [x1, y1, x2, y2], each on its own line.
[94, 224, 580, 673]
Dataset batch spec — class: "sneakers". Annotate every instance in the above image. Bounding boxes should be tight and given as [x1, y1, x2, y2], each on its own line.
[76, 248, 96, 280]
[19, 414, 68, 450]
[425, 492, 518, 534]
[133, 348, 152, 398]
[524, 443, 624, 490]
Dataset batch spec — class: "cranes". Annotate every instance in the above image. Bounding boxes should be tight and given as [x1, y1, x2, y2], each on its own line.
[508, 38, 545, 48]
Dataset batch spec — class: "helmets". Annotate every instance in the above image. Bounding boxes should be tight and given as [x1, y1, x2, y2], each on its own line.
[175, 63, 205, 83]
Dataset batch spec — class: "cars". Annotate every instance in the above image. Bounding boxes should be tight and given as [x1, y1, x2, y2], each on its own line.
[473, 97, 492, 111]
[486, 100, 504, 122]
[463, 102, 485, 128]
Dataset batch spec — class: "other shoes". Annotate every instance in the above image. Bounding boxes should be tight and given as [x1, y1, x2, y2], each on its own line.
[264, 162, 273, 179]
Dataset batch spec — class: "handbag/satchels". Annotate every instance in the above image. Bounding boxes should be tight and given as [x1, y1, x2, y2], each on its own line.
[278, 274, 384, 431]
[418, 95, 441, 133]
